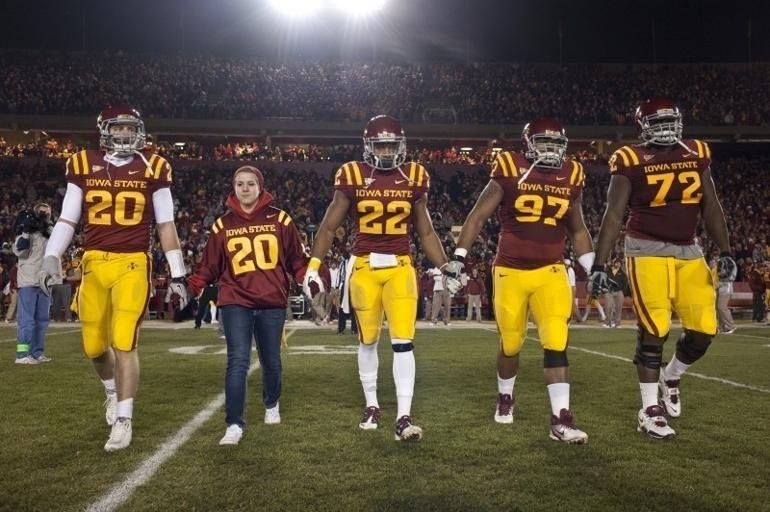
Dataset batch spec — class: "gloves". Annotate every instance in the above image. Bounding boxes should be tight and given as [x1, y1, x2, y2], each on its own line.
[303, 257, 324, 299]
[443, 256, 468, 291]
[165, 277, 188, 310]
[39, 256, 61, 296]
[718, 256, 737, 282]
[587, 265, 607, 299]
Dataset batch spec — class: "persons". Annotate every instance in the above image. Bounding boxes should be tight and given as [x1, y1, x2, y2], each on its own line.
[0, 49, 770, 337]
[584, 94, 740, 441]
[440, 115, 610, 447]
[167, 164, 332, 446]
[300, 114, 468, 441]
[9, 203, 54, 365]
[41, 101, 194, 455]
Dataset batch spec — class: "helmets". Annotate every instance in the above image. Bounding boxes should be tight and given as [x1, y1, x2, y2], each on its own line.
[362, 115, 407, 170]
[522, 118, 569, 170]
[636, 98, 683, 147]
[96, 104, 145, 155]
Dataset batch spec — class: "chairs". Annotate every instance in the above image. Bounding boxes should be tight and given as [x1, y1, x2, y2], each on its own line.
[479, 297, 490, 317]
[450, 296, 468, 317]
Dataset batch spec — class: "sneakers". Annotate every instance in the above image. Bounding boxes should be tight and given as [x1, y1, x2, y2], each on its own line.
[637, 405, 675, 439]
[549, 408, 588, 444]
[359, 406, 380, 430]
[219, 424, 243, 445]
[264, 401, 281, 425]
[104, 419, 132, 451]
[14, 356, 38, 365]
[494, 393, 515, 423]
[36, 356, 51, 362]
[659, 362, 681, 418]
[395, 415, 422, 441]
[104, 392, 118, 425]
